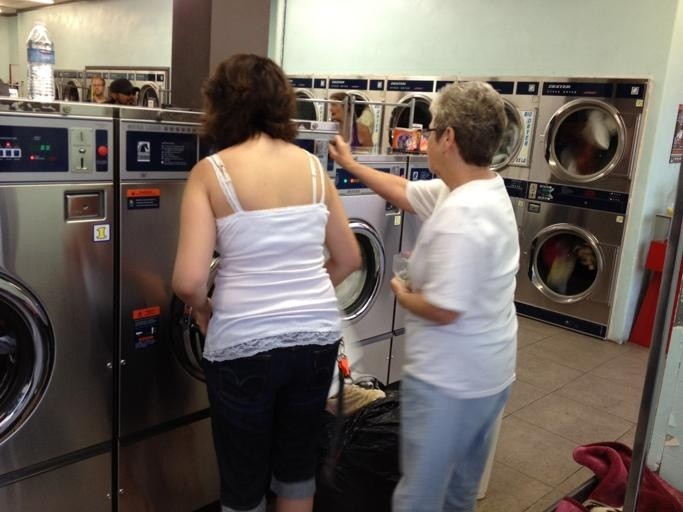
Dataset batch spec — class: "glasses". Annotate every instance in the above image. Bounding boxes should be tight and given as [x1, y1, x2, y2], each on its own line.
[126, 92, 136, 96]
[421, 128, 436, 137]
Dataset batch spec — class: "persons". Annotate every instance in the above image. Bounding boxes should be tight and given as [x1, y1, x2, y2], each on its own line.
[171, 52, 362, 512]
[330, 92, 372, 147]
[329, 80, 522, 512]
[86, 75, 140, 106]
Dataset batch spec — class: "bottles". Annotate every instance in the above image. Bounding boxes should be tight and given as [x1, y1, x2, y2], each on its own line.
[26, 20, 56, 101]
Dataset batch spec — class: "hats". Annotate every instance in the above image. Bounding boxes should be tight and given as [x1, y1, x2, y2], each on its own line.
[110, 78, 141, 94]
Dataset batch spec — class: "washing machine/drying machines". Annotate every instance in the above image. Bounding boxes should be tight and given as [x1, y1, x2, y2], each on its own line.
[342, 144, 510, 502]
[6, 62, 172, 109]
[285, 63, 655, 345]
[2, 90, 342, 512]
